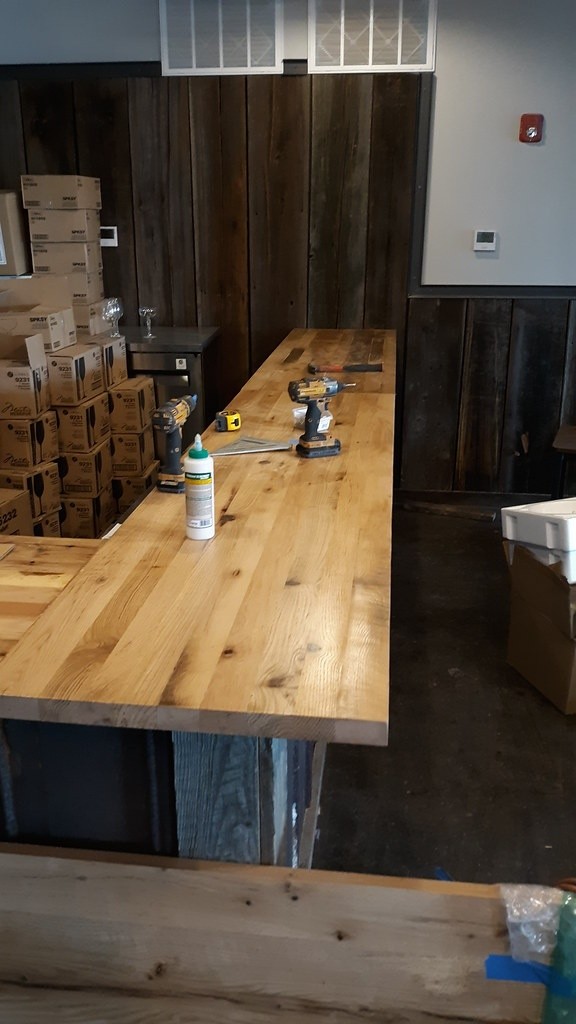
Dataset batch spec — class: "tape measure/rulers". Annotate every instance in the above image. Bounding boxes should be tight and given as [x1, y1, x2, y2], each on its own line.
[214, 409, 241, 432]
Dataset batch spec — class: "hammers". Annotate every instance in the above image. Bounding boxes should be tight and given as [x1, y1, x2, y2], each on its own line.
[308, 363, 383, 375]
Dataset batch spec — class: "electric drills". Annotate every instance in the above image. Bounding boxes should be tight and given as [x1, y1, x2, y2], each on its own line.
[151, 394, 198, 493]
[287, 376, 356, 458]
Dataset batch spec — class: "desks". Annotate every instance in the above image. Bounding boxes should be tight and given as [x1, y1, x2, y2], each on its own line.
[0, 329, 399, 868]
[117, 325, 220, 440]
[0, 533, 173, 857]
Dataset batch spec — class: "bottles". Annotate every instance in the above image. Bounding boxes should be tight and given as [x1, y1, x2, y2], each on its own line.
[184, 434, 215, 540]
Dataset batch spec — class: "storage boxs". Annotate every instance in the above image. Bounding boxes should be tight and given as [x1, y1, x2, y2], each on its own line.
[502, 537, 576, 719]
[0, 172, 161, 539]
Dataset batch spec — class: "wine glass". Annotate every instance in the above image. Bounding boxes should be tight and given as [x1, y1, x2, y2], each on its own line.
[102, 298, 123, 338]
[139, 306, 157, 339]
